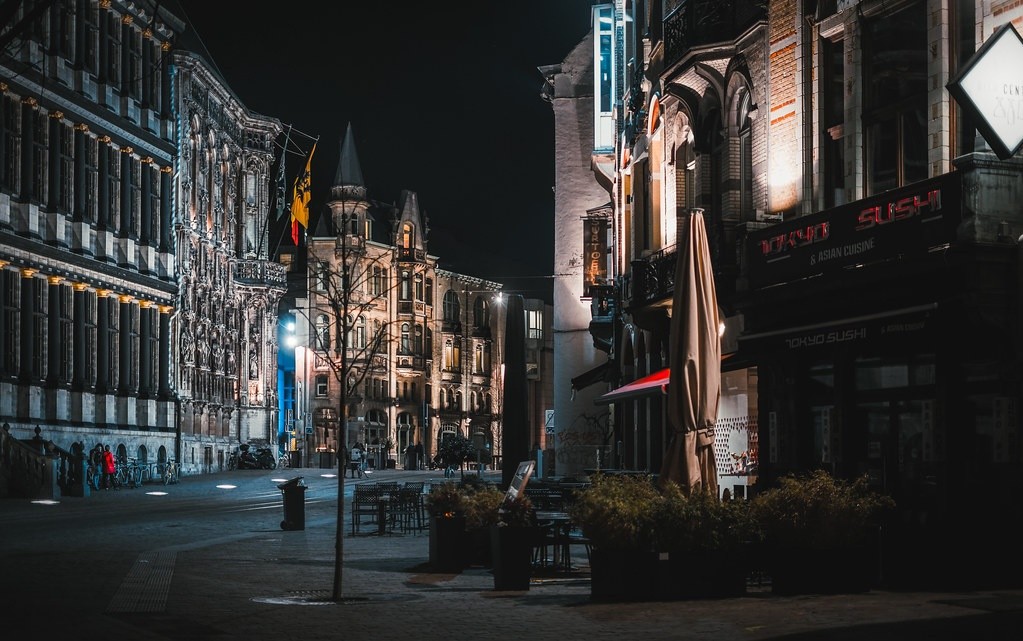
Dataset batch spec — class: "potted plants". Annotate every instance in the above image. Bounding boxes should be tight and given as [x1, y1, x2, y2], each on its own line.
[567, 473, 898, 604]
[425, 481, 538, 593]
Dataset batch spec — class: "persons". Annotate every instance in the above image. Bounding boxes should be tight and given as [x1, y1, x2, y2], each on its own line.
[92, 445, 103, 490]
[101, 445, 119, 490]
[406, 441, 424, 471]
[336, 442, 369, 479]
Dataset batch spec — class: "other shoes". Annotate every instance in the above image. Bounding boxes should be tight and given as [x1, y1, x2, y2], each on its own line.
[105, 487, 109, 491]
[115, 487, 120, 491]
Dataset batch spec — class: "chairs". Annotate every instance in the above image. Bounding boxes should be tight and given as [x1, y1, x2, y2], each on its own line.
[352, 480, 429, 537]
[522, 484, 592, 573]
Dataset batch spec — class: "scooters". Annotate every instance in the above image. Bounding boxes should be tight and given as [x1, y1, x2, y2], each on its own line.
[224, 443, 277, 471]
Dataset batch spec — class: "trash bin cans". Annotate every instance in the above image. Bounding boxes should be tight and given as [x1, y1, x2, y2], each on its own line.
[289, 451, 302, 468]
[368, 458, 374, 468]
[387, 459, 396, 469]
[277, 476, 308, 530]
[320, 452, 333, 469]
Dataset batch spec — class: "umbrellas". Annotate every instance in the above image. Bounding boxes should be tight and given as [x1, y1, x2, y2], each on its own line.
[657, 207, 721, 500]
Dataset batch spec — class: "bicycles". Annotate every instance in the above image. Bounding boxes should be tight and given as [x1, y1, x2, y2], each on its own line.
[88, 453, 176, 491]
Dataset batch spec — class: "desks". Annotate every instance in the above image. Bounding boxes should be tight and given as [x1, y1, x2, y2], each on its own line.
[535, 512, 573, 574]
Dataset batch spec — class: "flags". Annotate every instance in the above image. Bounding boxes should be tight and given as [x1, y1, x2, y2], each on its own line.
[272, 154, 287, 221]
[290, 143, 317, 246]
